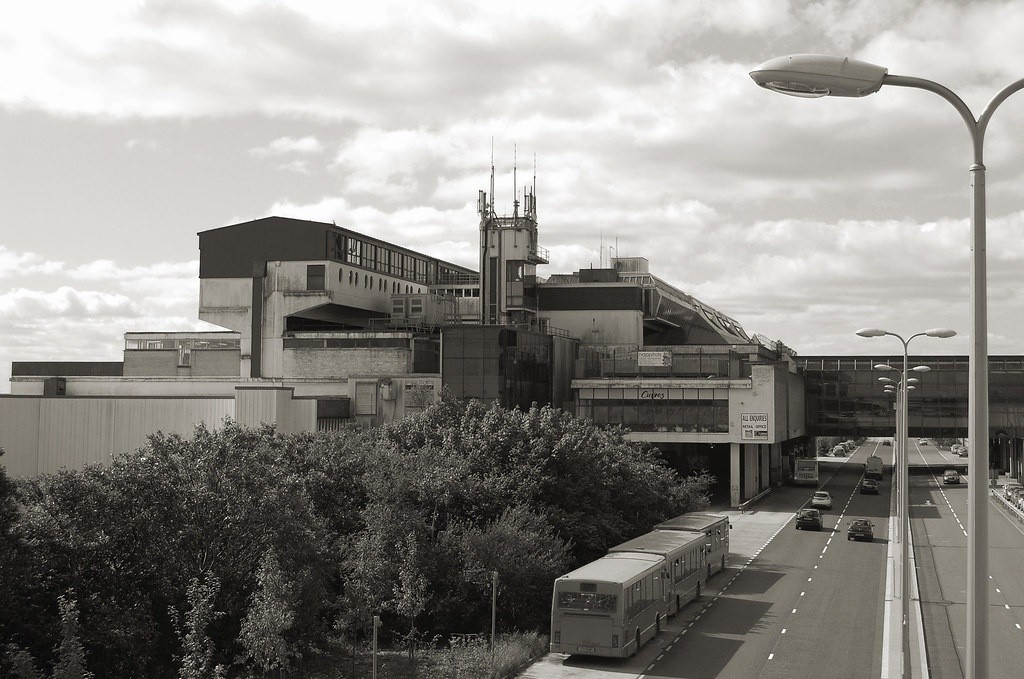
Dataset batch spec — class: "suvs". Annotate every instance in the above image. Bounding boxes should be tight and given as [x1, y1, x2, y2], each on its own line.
[795, 509, 824, 531]
[859, 478, 880, 496]
[811, 492, 834, 509]
[847, 518, 876, 542]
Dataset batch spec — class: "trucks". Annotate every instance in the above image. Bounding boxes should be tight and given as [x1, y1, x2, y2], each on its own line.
[863, 456, 884, 479]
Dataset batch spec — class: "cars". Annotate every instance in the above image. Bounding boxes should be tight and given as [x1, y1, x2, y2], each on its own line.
[919, 439, 928, 446]
[882, 440, 891, 446]
[708, 375, 716, 379]
[950, 444, 960, 454]
[958, 447, 968, 458]
[749, 375, 752, 380]
[832, 439, 855, 457]
[940, 470, 960, 484]
[1002, 482, 1024, 514]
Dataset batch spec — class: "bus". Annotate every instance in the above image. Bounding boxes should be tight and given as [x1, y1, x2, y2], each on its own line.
[549, 552, 671, 659]
[607, 528, 707, 618]
[653, 512, 733, 581]
[793, 456, 819, 485]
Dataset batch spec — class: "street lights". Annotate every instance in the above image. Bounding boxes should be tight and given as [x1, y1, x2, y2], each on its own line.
[748, 54, 1024, 678]
[846, 328, 957, 679]
